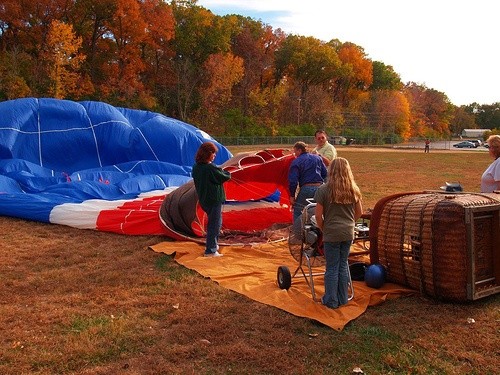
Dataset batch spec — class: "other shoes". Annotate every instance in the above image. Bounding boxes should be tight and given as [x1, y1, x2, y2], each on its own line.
[205, 251, 223, 256]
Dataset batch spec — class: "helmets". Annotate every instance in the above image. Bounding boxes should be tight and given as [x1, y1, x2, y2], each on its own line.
[365, 263, 386, 287]
[350, 262, 368, 281]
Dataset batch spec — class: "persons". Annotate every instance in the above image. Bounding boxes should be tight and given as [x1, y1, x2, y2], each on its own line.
[314, 157, 363, 309]
[192, 142, 231, 256]
[289, 142, 328, 245]
[481, 134, 500, 192]
[425, 138, 430, 153]
[311, 129, 337, 172]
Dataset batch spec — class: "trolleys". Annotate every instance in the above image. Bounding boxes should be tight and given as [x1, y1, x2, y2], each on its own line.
[277, 198, 354, 305]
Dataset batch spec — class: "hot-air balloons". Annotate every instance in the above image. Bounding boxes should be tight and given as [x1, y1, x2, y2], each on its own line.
[0, 97, 500, 301]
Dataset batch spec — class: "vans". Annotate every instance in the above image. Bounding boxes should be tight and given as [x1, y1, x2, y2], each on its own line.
[329, 136, 346, 145]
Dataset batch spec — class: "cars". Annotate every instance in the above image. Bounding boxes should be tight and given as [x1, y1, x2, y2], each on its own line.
[453, 138, 489, 148]
